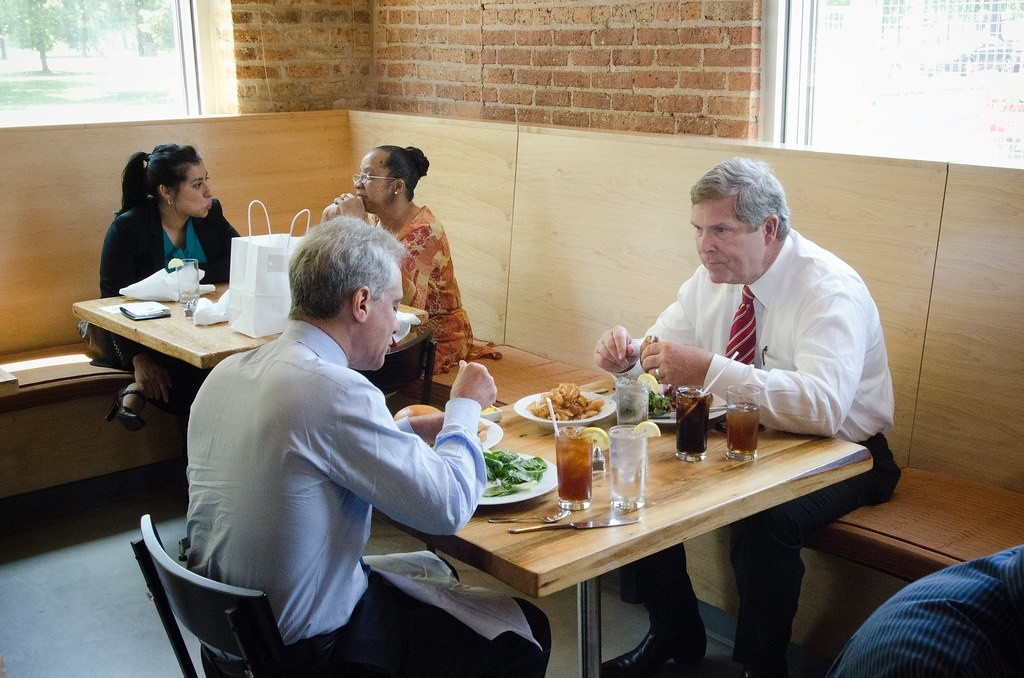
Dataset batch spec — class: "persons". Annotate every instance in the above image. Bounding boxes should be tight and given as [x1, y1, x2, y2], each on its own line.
[100, 144, 241, 431]
[322, 144, 502, 403]
[595, 159, 902, 678]
[187, 217, 551, 678]
[825, 545, 1024, 678]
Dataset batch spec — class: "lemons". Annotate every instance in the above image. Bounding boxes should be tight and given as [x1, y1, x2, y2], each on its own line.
[638, 374, 659, 393]
[634, 421, 661, 437]
[582, 427, 609, 446]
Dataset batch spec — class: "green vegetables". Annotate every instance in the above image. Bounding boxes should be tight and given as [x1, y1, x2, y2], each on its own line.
[646, 392, 672, 419]
[479, 448, 549, 497]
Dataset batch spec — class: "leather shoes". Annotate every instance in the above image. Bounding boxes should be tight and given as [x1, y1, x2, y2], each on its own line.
[600, 616, 707, 678]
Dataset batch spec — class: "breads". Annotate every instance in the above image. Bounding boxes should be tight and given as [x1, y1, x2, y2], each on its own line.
[393, 404, 443, 421]
[640, 335, 659, 358]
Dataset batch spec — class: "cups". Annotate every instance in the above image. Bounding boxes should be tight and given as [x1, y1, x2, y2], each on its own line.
[726, 384, 762, 461]
[615, 379, 650, 426]
[608, 425, 649, 515]
[675, 385, 712, 463]
[553, 425, 594, 511]
[176, 258, 199, 319]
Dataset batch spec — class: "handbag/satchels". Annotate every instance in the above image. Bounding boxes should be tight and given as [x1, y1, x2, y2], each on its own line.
[228, 199, 311, 337]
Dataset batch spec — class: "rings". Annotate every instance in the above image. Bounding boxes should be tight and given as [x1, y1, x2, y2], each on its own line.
[656, 368, 662, 382]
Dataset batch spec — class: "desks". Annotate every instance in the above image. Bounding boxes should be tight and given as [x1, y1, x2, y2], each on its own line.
[70, 283, 435, 412]
[394, 379, 872, 678]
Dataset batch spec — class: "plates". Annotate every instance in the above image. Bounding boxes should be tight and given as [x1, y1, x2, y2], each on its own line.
[611, 392, 729, 424]
[478, 452, 559, 505]
[479, 418, 504, 450]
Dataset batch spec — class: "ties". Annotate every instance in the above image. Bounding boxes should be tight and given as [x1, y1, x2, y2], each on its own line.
[725, 285, 757, 366]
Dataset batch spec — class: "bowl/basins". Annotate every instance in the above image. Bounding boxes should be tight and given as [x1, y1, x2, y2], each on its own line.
[514, 390, 617, 430]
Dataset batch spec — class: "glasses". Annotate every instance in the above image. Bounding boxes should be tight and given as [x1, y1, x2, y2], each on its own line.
[352, 173, 395, 183]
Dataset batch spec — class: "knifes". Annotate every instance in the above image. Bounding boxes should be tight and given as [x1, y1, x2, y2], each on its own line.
[509, 516, 642, 535]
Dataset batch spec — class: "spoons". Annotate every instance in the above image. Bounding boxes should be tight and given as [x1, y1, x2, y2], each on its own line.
[488, 511, 572, 524]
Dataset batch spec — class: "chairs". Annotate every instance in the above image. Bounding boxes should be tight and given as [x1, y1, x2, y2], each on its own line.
[128, 514, 297, 678]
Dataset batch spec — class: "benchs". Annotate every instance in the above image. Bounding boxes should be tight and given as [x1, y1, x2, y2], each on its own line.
[0, 112, 1024, 578]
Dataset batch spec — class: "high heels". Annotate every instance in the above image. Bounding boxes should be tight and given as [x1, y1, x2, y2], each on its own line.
[104, 385, 148, 431]
